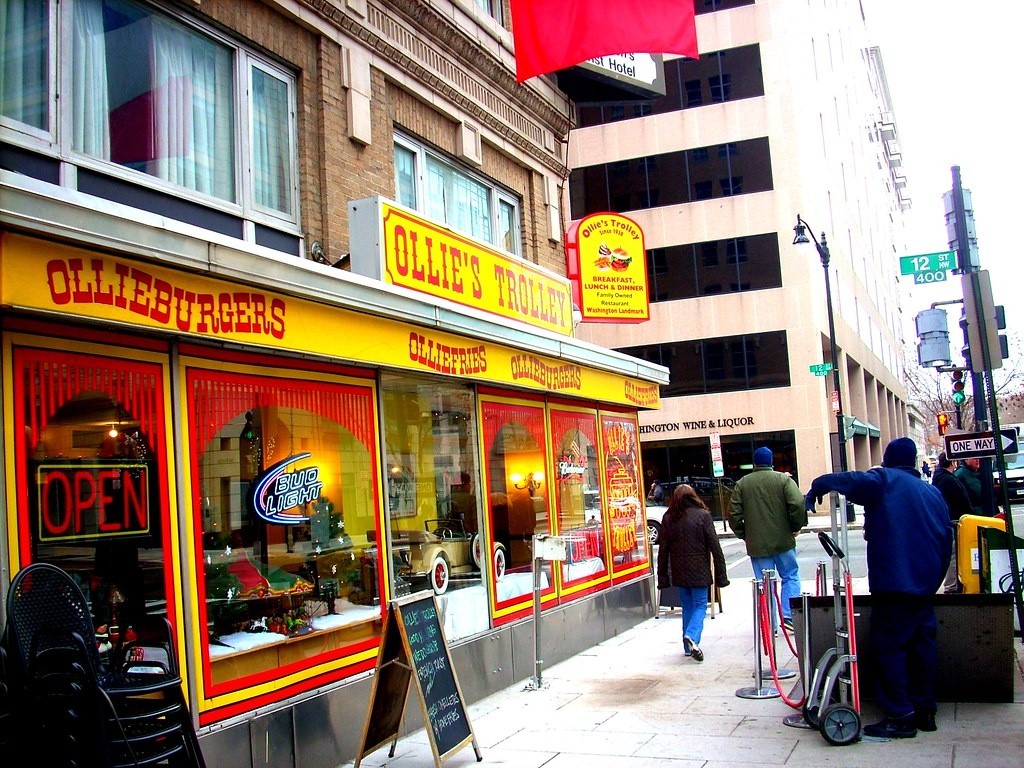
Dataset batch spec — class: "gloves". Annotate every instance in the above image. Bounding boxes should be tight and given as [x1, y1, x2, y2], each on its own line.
[805, 489, 823, 514]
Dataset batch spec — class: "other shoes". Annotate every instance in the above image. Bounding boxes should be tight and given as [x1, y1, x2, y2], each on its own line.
[779, 618, 794, 631]
[864, 718, 918, 738]
[917, 714, 937, 732]
[775, 631, 778, 637]
[683, 636, 704, 661]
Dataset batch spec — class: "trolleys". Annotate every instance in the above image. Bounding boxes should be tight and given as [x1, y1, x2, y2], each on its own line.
[802, 531, 862, 746]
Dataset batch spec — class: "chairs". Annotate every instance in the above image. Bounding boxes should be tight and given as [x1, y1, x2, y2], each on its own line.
[1, 562, 207, 768]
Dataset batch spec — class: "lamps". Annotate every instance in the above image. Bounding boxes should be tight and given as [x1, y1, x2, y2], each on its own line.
[511, 472, 545, 496]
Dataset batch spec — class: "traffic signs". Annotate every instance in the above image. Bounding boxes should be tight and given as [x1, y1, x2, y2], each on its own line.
[944, 428, 1019, 461]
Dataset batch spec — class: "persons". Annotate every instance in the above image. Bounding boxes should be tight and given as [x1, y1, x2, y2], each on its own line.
[652, 484, 731, 662]
[644, 469, 657, 495]
[438, 462, 531, 535]
[725, 446, 809, 639]
[647, 480, 664, 505]
[917, 449, 1009, 596]
[803, 437, 954, 739]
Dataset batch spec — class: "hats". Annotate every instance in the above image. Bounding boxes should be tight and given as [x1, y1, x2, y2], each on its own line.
[881, 437, 917, 468]
[753, 447, 773, 467]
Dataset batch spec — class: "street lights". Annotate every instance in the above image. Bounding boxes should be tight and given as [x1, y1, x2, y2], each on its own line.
[792, 213, 856, 522]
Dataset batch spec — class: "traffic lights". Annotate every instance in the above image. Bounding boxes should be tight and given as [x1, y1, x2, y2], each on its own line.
[951, 370, 965, 405]
[937, 414, 948, 436]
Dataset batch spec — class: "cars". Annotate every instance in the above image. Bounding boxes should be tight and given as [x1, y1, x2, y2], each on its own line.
[991, 441, 1024, 502]
[392, 516, 506, 596]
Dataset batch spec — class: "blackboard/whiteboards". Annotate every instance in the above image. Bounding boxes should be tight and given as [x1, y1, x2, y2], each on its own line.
[359, 589, 474, 763]
[657, 551, 721, 608]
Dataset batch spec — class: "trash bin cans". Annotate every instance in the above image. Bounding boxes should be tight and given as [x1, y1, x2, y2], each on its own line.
[846, 500, 855, 522]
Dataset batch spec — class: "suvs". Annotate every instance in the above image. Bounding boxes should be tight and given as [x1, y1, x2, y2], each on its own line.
[583, 490, 670, 544]
[660, 477, 739, 516]
